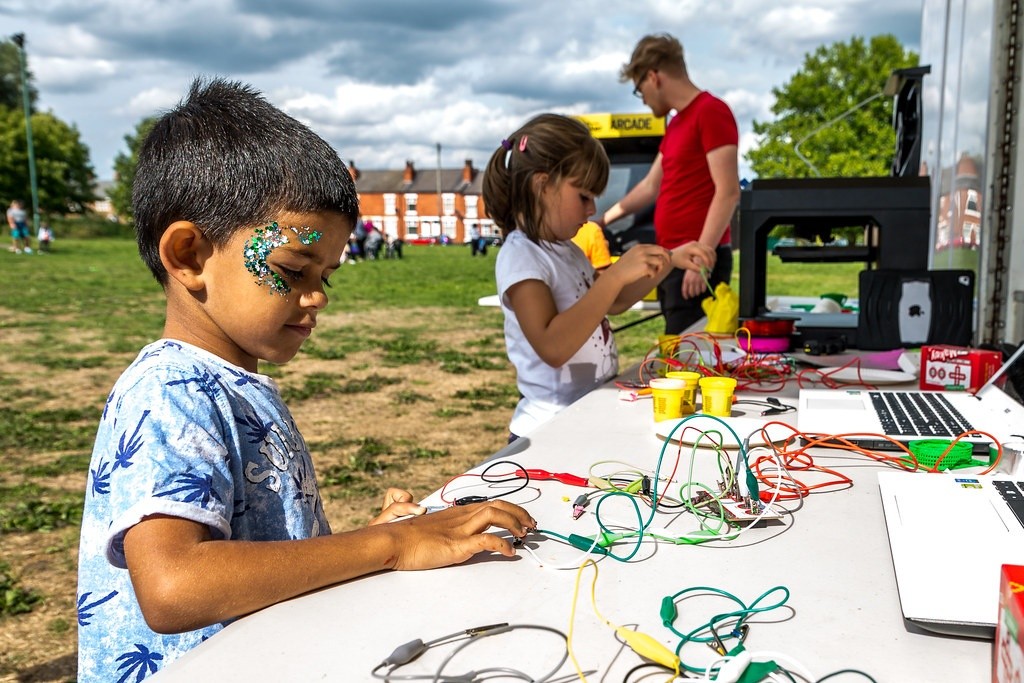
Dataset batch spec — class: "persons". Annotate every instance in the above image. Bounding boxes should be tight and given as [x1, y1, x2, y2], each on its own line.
[471, 224, 479, 256]
[340, 215, 403, 266]
[78, 83, 536, 682]
[7, 200, 53, 254]
[592, 32, 742, 335]
[482, 113, 718, 444]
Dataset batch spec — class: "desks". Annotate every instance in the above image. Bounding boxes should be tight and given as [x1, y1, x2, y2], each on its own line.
[138, 295, 1023, 683]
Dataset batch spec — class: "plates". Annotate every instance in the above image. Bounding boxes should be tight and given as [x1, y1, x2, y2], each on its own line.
[817, 367, 916, 385]
[656, 416, 796, 449]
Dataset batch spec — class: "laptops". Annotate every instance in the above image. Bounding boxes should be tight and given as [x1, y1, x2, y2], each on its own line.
[799, 344, 1024, 451]
[855, 269, 976, 351]
[876, 469, 1024, 638]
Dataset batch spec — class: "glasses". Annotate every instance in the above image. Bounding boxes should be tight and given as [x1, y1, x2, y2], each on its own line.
[633, 67, 658, 98]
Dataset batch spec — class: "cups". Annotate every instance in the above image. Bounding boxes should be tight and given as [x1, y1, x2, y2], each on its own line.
[649, 378, 686, 422]
[699, 376, 738, 417]
[665, 370, 701, 415]
